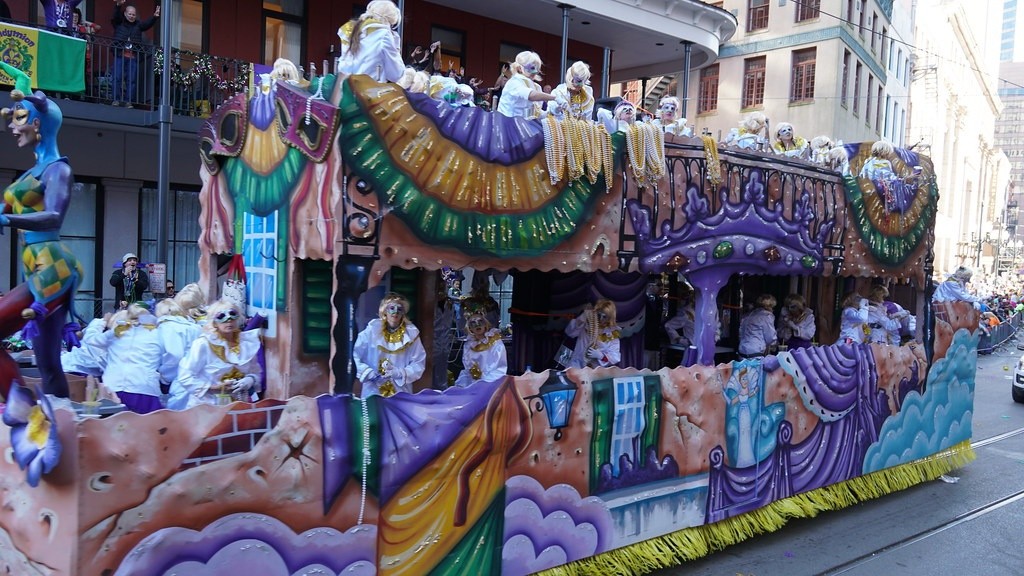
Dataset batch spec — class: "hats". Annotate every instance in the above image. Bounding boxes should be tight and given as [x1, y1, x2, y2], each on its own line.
[122, 253, 138, 266]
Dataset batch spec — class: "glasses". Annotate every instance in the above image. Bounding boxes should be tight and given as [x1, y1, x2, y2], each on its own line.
[166, 286, 174, 289]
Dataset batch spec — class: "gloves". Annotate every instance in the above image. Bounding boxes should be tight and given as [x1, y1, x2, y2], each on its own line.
[577, 309, 592, 321]
[366, 371, 379, 380]
[977, 297, 984, 303]
[787, 320, 795, 329]
[587, 347, 604, 359]
[554, 94, 568, 110]
[231, 374, 258, 394]
[381, 368, 399, 379]
[859, 299, 868, 308]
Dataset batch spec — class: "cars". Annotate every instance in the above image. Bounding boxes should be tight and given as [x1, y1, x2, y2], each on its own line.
[1012, 344, 1024, 403]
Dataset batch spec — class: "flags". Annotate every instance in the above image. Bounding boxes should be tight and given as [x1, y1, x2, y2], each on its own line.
[0, 22, 86, 95]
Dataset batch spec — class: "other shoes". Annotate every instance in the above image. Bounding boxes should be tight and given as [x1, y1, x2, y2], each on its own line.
[79, 97, 85, 101]
[124, 102, 134, 109]
[112, 101, 120, 106]
[65, 95, 72, 100]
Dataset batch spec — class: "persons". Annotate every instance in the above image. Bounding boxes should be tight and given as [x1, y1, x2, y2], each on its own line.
[60, 252, 264, 412]
[0, 61, 85, 404]
[111, 0, 160, 110]
[353, 292, 427, 399]
[261, 0, 921, 183]
[39, 0, 102, 100]
[440, 265, 1024, 387]
